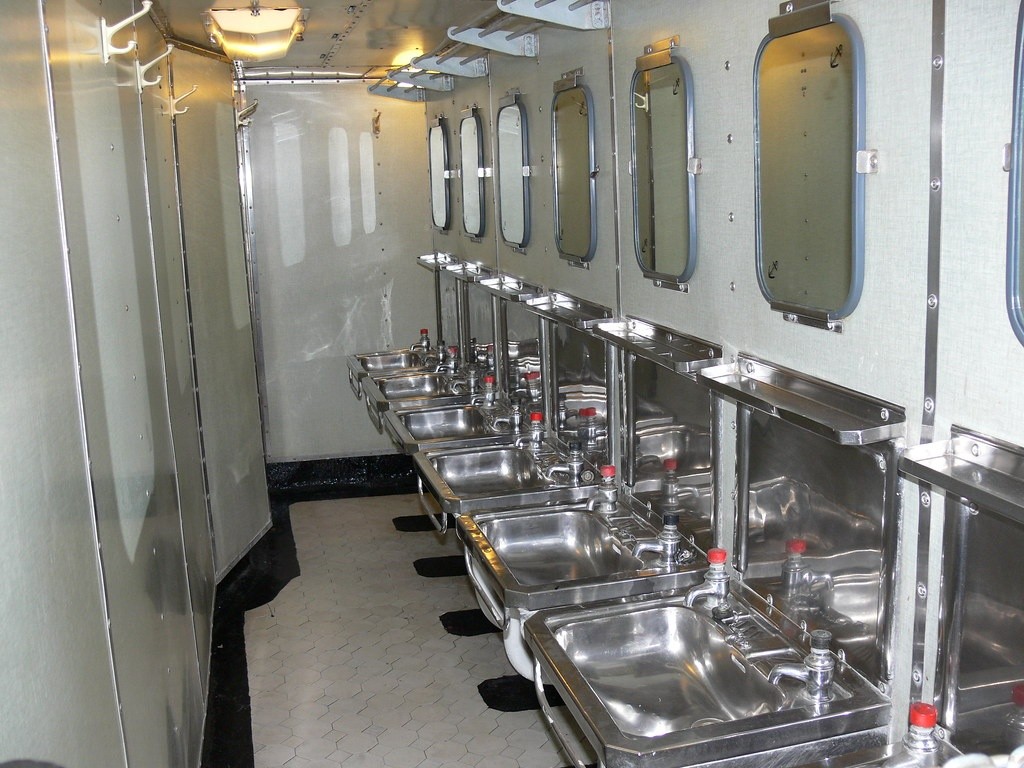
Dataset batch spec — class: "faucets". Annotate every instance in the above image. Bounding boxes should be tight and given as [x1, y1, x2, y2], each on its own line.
[435, 348, 457, 373]
[470, 337, 486, 362]
[559, 393, 578, 429]
[410, 329, 429, 354]
[515, 412, 543, 450]
[683, 548, 730, 610]
[450, 371, 477, 395]
[662, 459, 699, 513]
[579, 407, 608, 446]
[545, 442, 583, 483]
[586, 466, 617, 513]
[471, 376, 495, 408]
[487, 345, 494, 366]
[767, 630, 834, 703]
[420, 340, 445, 363]
[780, 538, 834, 603]
[493, 405, 522, 431]
[508, 359, 524, 388]
[634, 436, 661, 469]
[632, 514, 681, 567]
[880, 700, 939, 768]
[525, 371, 540, 402]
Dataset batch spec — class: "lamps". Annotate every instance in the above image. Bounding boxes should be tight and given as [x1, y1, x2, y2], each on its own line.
[199, 7, 310, 63]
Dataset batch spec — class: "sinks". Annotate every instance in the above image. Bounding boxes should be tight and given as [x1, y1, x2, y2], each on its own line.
[361, 374, 476, 412]
[456, 495, 708, 608]
[570, 425, 712, 493]
[670, 479, 885, 564]
[382, 404, 523, 454]
[412, 443, 602, 513]
[528, 387, 673, 437]
[752, 567, 1024, 693]
[524, 584, 892, 768]
[346, 348, 436, 382]
[599, 422, 754, 490]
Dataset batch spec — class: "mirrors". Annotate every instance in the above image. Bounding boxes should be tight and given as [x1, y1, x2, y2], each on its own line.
[428, 112, 451, 236]
[551, 66, 600, 271]
[1002, 0, 1024, 347]
[628, 34, 703, 293]
[752, 0, 880, 334]
[459, 102, 485, 245]
[497, 87, 530, 256]
